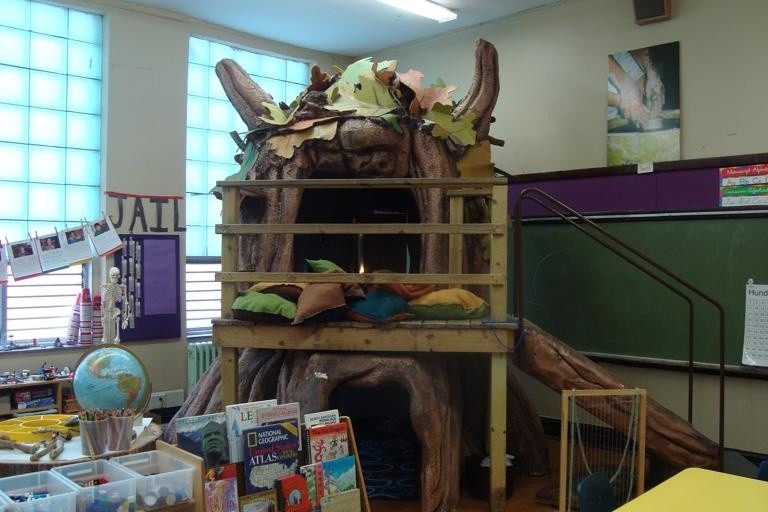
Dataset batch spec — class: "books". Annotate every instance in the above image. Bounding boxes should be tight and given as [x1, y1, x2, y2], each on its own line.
[0, 216, 124, 284]
[176, 399, 362, 512]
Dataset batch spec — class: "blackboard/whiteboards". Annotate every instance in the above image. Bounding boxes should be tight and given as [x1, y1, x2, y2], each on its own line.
[507, 211, 768, 380]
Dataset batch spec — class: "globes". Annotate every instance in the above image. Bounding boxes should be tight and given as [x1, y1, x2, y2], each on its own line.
[72, 342, 153, 441]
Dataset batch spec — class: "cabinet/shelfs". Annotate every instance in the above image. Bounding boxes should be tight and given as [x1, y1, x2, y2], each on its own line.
[0, 373, 81, 421]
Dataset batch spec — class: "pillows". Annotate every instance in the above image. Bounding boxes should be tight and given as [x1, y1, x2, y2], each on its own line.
[229, 256, 491, 325]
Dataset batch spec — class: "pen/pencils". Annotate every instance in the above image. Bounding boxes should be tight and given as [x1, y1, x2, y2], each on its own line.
[78, 407, 134, 457]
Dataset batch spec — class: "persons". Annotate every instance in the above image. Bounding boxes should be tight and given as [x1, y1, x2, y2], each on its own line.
[101, 267, 128, 345]
[608, 49, 666, 131]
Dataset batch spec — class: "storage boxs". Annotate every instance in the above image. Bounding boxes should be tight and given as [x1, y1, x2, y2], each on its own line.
[0, 448, 197, 512]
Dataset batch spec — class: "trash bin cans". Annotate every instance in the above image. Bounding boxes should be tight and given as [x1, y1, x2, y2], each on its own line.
[465, 454, 516, 501]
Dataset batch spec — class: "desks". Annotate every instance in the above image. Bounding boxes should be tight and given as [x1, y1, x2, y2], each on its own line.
[610, 467, 768, 512]
[0, 416, 163, 471]
